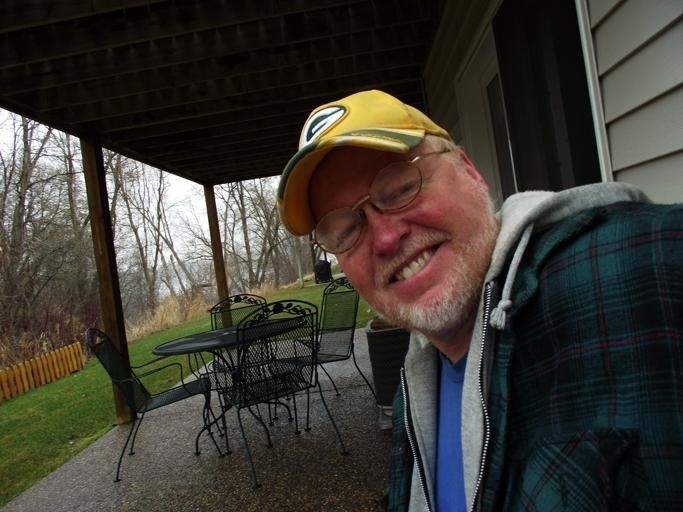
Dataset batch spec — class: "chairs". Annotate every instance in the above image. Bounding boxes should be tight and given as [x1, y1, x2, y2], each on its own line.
[210, 294, 269, 364]
[84, 327, 231, 483]
[219, 300, 348, 490]
[279, 276, 377, 431]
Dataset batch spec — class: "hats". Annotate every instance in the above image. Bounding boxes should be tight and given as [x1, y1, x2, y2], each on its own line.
[276, 90, 455, 237]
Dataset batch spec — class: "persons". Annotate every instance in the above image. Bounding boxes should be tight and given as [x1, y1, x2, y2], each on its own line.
[277, 89, 683, 512]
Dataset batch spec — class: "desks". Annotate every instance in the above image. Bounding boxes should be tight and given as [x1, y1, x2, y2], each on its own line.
[152, 318, 309, 456]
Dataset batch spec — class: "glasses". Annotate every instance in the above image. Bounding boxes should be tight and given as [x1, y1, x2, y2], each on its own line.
[309, 149, 452, 254]
